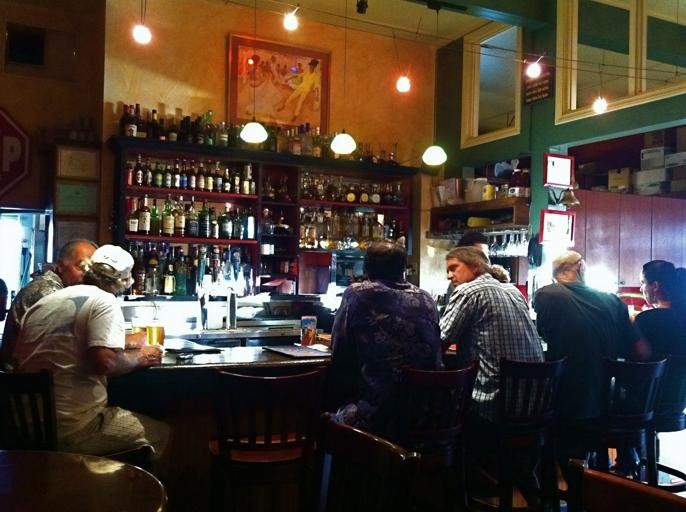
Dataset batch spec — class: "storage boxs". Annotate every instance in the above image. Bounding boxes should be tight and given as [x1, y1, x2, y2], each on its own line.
[608, 147, 686, 194]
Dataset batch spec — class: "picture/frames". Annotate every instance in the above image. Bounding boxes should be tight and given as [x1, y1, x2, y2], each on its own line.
[226, 32, 332, 137]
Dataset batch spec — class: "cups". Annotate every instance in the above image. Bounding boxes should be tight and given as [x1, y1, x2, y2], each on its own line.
[301, 314, 317, 346]
[131, 317, 148, 345]
[145, 320, 165, 347]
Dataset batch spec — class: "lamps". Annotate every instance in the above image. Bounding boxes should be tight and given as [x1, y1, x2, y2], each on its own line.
[543, 152, 576, 188]
[392, 17, 422, 93]
[593, 63, 607, 114]
[283, 4, 300, 31]
[330, 0, 357, 154]
[539, 208, 576, 245]
[239, 0, 268, 144]
[132, 0, 152, 44]
[422, 0, 448, 167]
[521, 55, 544, 78]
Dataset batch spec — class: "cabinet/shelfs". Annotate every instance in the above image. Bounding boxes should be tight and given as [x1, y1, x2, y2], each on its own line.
[107, 135, 422, 301]
[571, 189, 686, 292]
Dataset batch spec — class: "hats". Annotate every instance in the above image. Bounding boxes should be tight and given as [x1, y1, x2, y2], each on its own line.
[89, 244, 135, 289]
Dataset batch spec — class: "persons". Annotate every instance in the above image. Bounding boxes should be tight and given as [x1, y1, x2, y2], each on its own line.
[532, 250, 653, 512]
[614, 259, 686, 477]
[445, 232, 490, 307]
[0, 238, 99, 373]
[438, 246, 553, 512]
[8, 244, 174, 481]
[320, 240, 451, 512]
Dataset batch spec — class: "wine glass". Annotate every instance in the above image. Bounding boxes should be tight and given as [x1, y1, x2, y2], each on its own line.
[425, 229, 530, 258]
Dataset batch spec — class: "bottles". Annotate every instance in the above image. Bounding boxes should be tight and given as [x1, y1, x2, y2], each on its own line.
[497, 168, 531, 198]
[119, 104, 401, 168]
[300, 171, 407, 204]
[123, 195, 257, 239]
[297, 206, 407, 252]
[125, 151, 255, 195]
[259, 170, 297, 276]
[123, 241, 254, 297]
